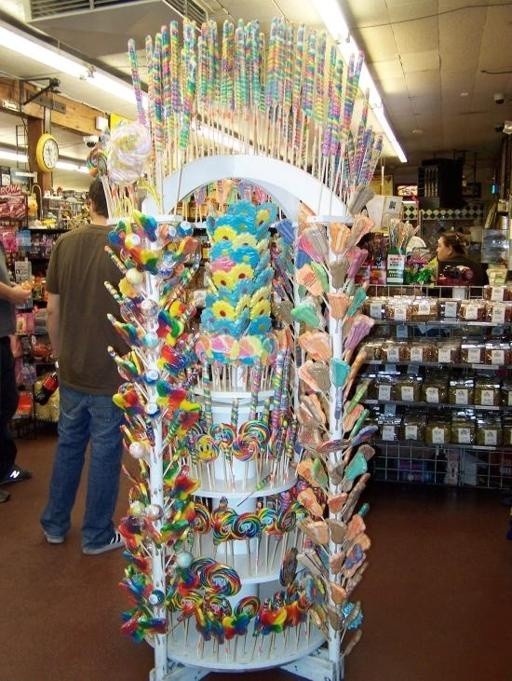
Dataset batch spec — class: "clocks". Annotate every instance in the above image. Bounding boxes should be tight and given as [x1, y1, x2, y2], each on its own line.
[35, 133, 60, 173]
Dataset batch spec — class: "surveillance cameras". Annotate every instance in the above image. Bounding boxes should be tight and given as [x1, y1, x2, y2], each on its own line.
[84, 135, 99, 148]
[494, 93, 505, 104]
[495, 125, 503, 132]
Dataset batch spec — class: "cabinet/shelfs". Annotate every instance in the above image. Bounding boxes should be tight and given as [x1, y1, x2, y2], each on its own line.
[138, 153, 355, 681]
[357, 319, 512, 452]
[0, 192, 88, 369]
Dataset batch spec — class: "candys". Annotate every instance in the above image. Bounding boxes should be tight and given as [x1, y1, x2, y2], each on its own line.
[87, 17, 385, 657]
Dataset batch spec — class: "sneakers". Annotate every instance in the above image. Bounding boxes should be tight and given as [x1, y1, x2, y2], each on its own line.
[0, 465, 32, 503]
[83, 529, 126, 555]
[41, 525, 65, 544]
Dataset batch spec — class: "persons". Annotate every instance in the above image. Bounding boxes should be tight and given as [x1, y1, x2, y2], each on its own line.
[38, 179, 133, 557]
[1, 234, 34, 504]
[435, 232, 484, 297]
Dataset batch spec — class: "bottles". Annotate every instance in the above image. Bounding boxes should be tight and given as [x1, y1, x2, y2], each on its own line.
[32, 371, 59, 406]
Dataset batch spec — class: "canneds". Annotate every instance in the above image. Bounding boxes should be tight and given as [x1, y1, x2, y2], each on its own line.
[499, 216, 508, 230]
